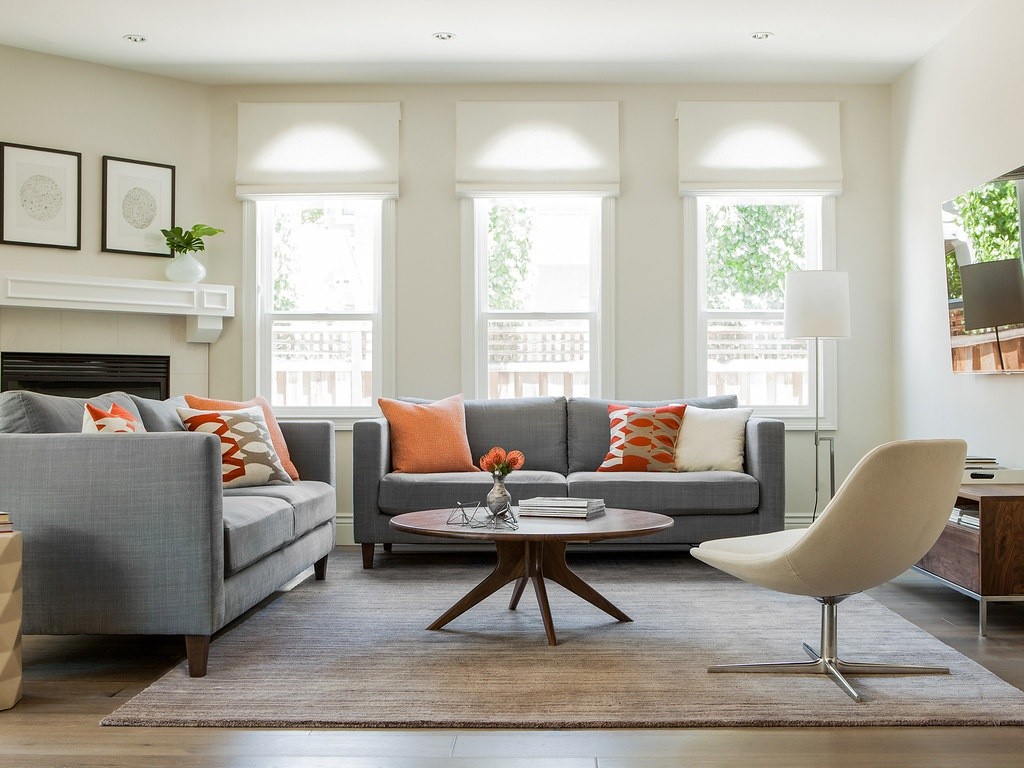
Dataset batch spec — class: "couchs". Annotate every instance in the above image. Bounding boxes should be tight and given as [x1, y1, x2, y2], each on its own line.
[354, 395, 786, 569]
[0, 390, 338, 678]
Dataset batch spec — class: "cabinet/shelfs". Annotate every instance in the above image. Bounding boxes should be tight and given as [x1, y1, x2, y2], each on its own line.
[912, 485, 1024, 638]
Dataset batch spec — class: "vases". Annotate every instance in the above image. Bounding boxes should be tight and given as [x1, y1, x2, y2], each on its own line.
[486, 476, 512, 512]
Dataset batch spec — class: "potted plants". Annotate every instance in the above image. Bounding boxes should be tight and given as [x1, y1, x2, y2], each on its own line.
[160, 223, 225, 284]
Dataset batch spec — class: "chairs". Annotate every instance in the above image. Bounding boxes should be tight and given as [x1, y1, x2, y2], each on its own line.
[688, 439, 968, 701]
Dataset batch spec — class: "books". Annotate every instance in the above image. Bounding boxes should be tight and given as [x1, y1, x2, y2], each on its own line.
[948, 503, 981, 530]
[964, 456, 1000, 469]
[519, 497, 606, 518]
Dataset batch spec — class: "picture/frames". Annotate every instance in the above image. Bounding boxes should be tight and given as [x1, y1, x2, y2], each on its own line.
[0, 143, 83, 251]
[102, 156, 176, 259]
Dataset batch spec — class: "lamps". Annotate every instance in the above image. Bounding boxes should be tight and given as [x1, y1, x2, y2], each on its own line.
[783, 271, 852, 522]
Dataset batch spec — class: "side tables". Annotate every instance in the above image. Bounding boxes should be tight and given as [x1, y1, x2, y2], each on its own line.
[0, 530, 25, 713]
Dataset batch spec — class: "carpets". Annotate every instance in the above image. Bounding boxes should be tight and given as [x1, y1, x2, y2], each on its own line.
[102, 543, 1023, 723]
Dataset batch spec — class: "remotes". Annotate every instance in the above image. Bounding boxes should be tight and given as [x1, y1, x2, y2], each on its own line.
[971, 472, 995, 479]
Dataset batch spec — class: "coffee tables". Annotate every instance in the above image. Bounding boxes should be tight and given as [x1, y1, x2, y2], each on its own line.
[390, 506, 674, 646]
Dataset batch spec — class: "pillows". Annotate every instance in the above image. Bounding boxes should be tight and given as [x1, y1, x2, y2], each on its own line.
[669, 404, 753, 472]
[82, 402, 146, 434]
[175, 406, 294, 489]
[596, 402, 686, 471]
[183, 393, 299, 480]
[376, 394, 480, 474]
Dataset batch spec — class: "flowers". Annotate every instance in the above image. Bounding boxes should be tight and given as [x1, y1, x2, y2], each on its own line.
[479, 445, 524, 479]
